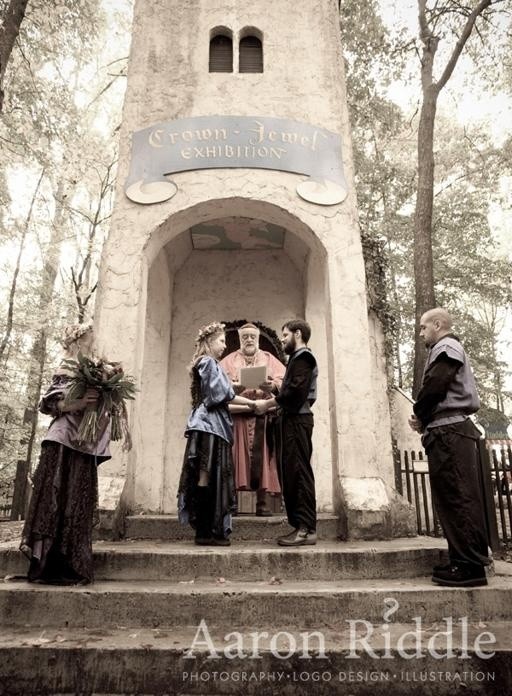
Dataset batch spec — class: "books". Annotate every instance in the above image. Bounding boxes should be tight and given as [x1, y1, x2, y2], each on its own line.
[238, 365, 267, 390]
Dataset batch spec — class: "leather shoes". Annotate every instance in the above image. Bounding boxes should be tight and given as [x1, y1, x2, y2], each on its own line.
[277, 523, 318, 546]
[256, 500, 273, 516]
[432, 560, 488, 586]
[195, 533, 231, 545]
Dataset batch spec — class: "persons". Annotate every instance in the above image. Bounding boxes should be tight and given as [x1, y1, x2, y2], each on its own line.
[210, 320, 288, 516]
[17, 321, 108, 587]
[407, 306, 493, 588]
[180, 320, 262, 547]
[245, 320, 319, 546]
